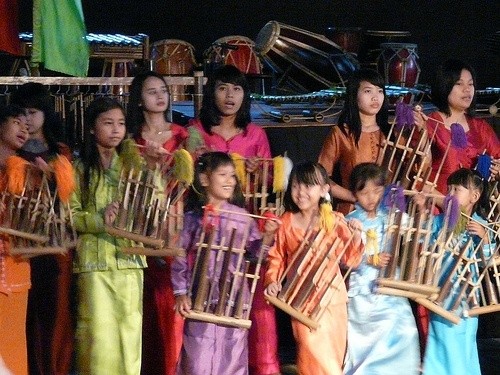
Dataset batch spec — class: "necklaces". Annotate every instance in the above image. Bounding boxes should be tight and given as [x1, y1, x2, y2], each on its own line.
[145, 123, 167, 135]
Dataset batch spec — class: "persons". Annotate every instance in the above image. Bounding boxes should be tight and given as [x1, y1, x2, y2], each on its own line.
[317, 67, 433, 219]
[262, 160, 365, 375]
[123, 72, 186, 375]
[61, 97, 156, 375]
[424, 55, 500, 230]
[8, 82, 73, 375]
[170, 152, 278, 375]
[341, 163, 424, 375]
[29, 0, 92, 79]
[185, 64, 283, 375]
[0, 106, 41, 375]
[421, 167, 499, 375]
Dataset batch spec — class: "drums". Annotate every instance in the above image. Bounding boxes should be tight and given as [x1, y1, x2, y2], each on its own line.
[362, 30, 411, 66]
[99, 58, 135, 104]
[254, 20, 359, 93]
[328, 26, 362, 54]
[148, 39, 197, 102]
[204, 35, 263, 75]
[376, 43, 421, 114]
